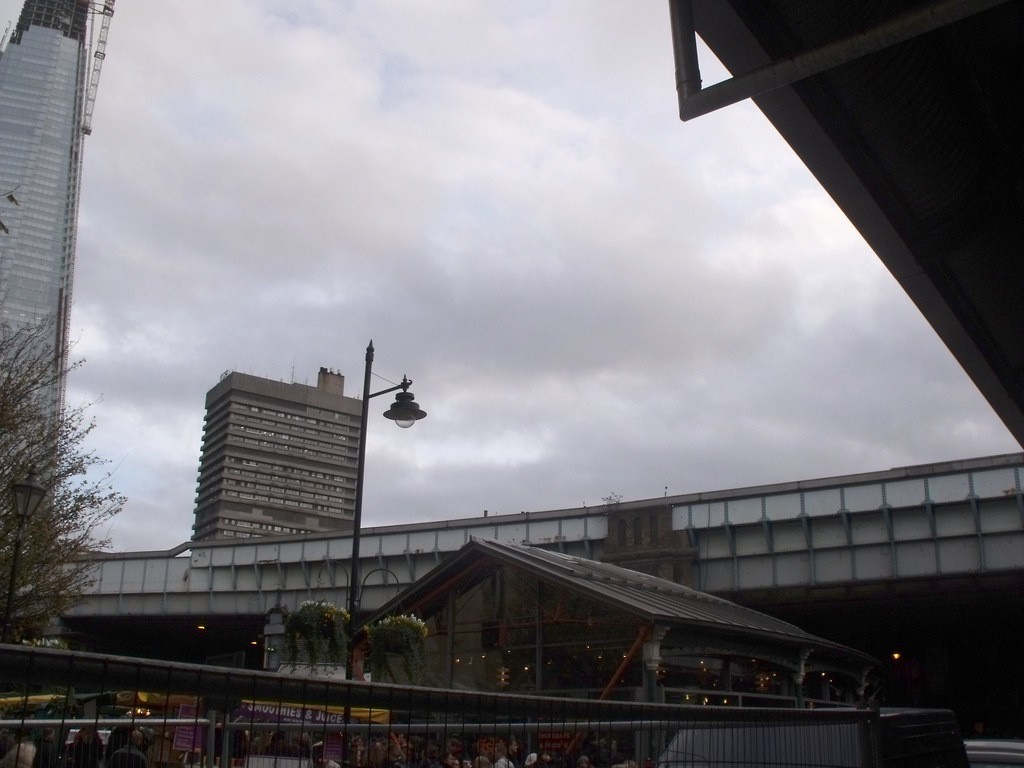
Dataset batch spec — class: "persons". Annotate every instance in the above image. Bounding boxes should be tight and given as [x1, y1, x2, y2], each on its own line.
[0, 715, 670, 768]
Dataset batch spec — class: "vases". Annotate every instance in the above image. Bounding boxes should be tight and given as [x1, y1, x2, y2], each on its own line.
[383, 633, 411, 652]
[298, 617, 334, 640]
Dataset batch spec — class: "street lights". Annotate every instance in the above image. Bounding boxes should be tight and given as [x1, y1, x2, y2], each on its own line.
[2, 462, 47, 641]
[340, 340, 427, 768]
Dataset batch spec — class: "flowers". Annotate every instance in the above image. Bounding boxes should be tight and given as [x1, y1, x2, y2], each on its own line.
[364, 614, 446, 686]
[285, 600, 351, 675]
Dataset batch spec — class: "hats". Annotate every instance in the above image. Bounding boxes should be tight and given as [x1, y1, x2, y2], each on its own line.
[525, 753, 538, 766]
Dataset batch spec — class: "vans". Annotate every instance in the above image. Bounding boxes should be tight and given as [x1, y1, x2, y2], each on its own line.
[656, 705, 971, 768]
